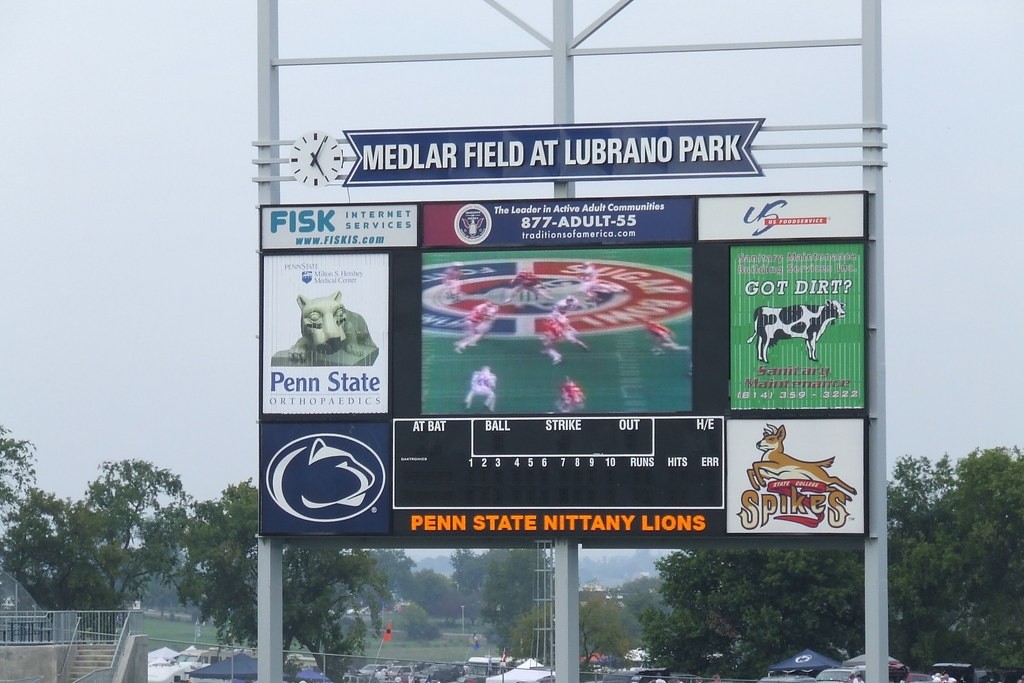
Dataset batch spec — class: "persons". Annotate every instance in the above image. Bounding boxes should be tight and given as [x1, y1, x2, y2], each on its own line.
[443, 260, 689, 413]
[649, 673, 721, 683]
[932, 673, 956, 683]
[849, 673, 864, 683]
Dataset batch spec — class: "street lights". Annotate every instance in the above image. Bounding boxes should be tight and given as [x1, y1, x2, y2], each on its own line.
[461, 606, 465, 634]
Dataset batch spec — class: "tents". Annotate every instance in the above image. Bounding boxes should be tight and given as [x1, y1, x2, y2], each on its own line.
[189, 653, 331, 683]
[768, 648, 841, 676]
[843, 654, 909, 666]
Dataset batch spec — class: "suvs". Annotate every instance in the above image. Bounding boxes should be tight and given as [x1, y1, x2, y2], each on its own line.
[356, 664, 390, 680]
[758, 667, 867, 683]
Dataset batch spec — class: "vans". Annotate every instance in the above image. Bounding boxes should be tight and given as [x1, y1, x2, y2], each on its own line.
[388, 665, 420, 683]
[171, 648, 229, 664]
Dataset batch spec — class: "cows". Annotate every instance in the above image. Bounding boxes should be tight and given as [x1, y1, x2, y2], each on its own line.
[745, 299, 846, 363]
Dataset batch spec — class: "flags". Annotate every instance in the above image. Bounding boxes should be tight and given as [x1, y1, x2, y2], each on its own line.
[383, 623, 394, 644]
[487, 652, 493, 672]
[471, 632, 480, 651]
[499, 648, 509, 662]
